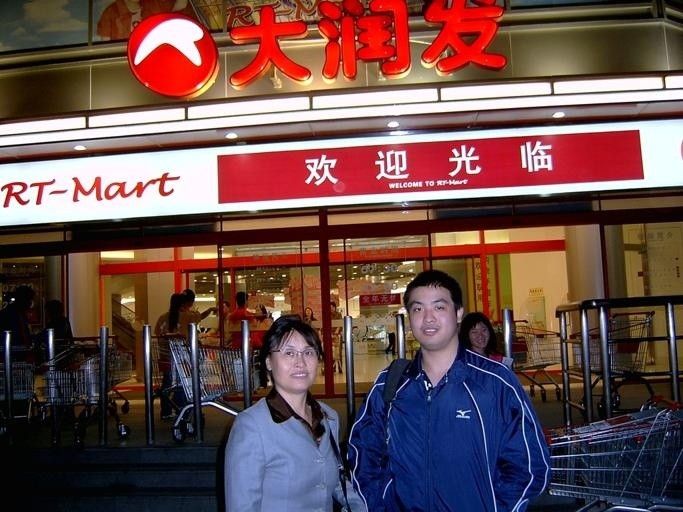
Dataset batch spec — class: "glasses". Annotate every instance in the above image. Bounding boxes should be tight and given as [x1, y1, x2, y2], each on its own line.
[269, 348, 319, 361]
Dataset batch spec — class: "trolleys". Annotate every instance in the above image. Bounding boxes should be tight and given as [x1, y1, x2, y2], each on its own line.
[0, 337, 47, 437]
[147, 335, 206, 426]
[44, 334, 134, 448]
[167, 332, 262, 443]
[569, 310, 659, 413]
[491, 318, 562, 404]
[541, 395, 682, 512]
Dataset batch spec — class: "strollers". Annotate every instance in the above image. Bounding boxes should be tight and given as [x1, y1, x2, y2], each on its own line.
[321, 334, 342, 376]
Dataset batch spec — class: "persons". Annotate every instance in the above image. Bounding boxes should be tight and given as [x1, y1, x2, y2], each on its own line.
[181, 288, 195, 311]
[153, 292, 218, 420]
[218, 291, 396, 375]
[458, 307, 514, 372]
[0, 285, 35, 364]
[36, 300, 76, 427]
[222, 312, 366, 511]
[342, 269, 551, 511]
